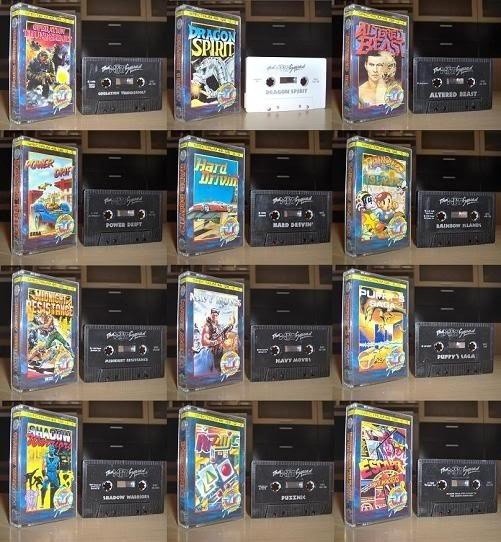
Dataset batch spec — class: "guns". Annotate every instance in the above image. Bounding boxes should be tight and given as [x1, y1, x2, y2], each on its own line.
[214, 323, 233, 345]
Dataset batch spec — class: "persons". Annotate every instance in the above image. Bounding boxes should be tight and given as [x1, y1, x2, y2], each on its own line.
[357, 49, 390, 107]
[34, 310, 74, 360]
[39, 443, 62, 509]
[24, 49, 55, 97]
[202, 307, 231, 374]
